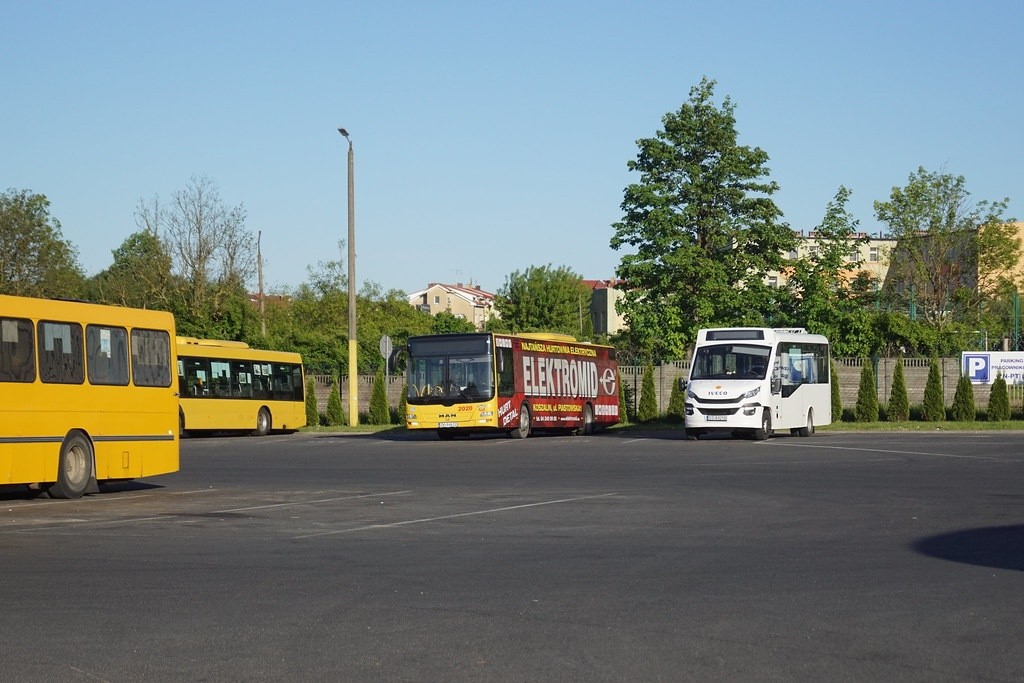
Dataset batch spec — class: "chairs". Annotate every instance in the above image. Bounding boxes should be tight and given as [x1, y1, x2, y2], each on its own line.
[749, 365, 765, 377]
[212, 375, 293, 397]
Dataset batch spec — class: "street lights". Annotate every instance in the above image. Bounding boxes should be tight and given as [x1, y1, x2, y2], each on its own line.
[335, 124, 362, 427]
[254, 230, 269, 345]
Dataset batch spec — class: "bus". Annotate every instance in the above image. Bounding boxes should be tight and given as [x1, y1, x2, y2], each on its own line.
[0, 290, 178, 496]
[682, 322, 834, 439]
[173, 334, 309, 439]
[399, 328, 622, 443]
